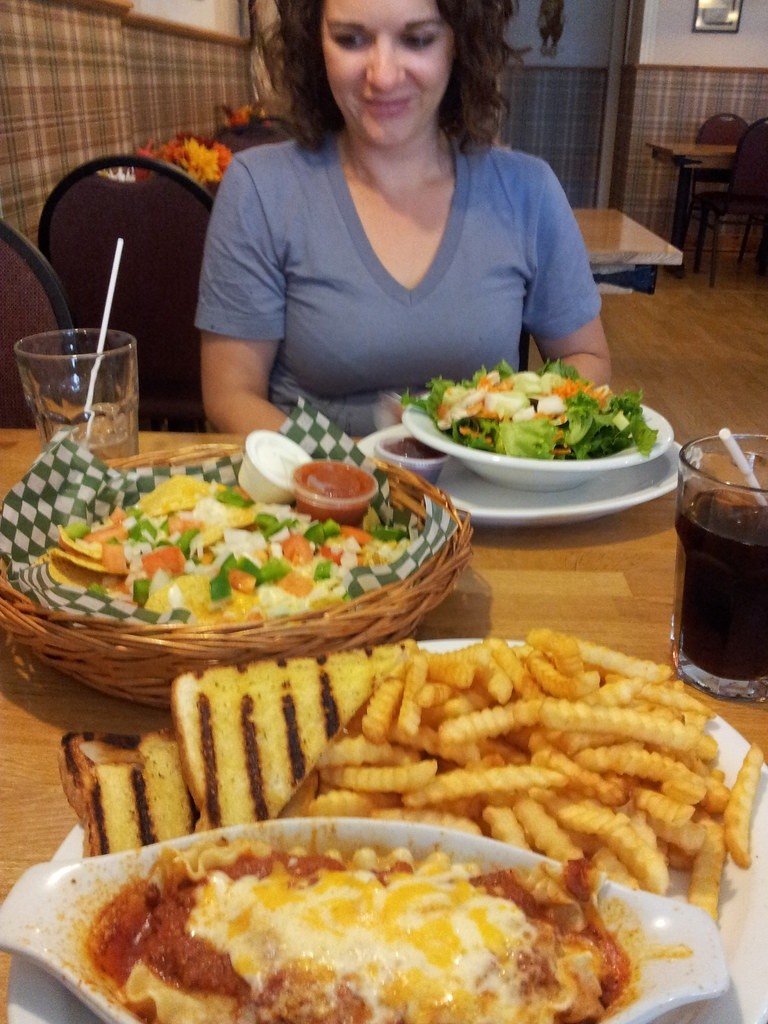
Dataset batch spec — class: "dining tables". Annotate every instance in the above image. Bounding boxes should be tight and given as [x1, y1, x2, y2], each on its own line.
[0, 430, 767, 1024]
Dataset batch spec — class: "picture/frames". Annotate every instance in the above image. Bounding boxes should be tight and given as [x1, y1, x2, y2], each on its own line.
[692, 0, 744, 33]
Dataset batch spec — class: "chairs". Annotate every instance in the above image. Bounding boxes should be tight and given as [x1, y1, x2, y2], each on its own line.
[0, 220, 76, 428]
[36, 153, 218, 434]
[680, 113, 754, 264]
[679, 116, 768, 288]
[214, 117, 295, 153]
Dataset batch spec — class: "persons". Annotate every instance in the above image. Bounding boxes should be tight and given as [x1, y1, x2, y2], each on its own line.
[193, 2, 613, 443]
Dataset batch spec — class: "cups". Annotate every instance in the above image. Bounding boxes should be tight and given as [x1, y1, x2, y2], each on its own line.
[674, 435, 768, 701]
[13, 328, 139, 462]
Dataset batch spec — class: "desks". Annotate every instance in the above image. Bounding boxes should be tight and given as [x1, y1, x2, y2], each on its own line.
[645, 141, 738, 281]
[519, 208, 684, 373]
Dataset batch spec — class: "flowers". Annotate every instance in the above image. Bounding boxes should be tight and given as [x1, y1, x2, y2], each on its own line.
[224, 101, 271, 129]
[132, 132, 232, 187]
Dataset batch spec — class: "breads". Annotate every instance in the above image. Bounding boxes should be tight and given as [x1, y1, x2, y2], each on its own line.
[56, 639, 416, 857]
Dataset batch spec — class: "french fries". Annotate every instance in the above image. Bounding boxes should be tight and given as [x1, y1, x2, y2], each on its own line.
[313, 630, 768, 927]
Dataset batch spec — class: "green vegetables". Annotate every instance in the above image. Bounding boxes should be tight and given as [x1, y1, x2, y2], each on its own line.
[395, 357, 657, 461]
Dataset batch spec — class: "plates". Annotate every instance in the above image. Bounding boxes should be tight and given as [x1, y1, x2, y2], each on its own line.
[0, 816, 731, 1024]
[0, 638, 768, 1024]
[356, 424, 700, 526]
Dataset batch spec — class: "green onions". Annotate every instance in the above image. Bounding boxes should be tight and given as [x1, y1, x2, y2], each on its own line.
[63, 486, 406, 605]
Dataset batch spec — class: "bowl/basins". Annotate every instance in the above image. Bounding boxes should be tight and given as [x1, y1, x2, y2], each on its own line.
[401, 392, 674, 490]
[238, 430, 312, 503]
[292, 461, 379, 525]
[374, 433, 449, 486]
